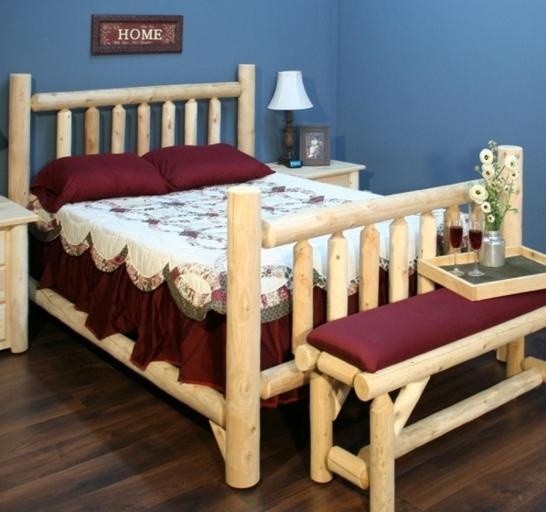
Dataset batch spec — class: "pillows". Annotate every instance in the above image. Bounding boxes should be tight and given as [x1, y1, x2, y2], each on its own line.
[29, 153, 176, 212]
[141, 141, 276, 193]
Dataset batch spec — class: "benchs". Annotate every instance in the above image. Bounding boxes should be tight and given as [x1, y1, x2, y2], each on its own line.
[291, 286, 546, 511]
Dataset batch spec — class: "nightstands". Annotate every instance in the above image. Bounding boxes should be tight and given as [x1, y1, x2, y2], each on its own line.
[0, 195, 43, 356]
[261, 157, 371, 192]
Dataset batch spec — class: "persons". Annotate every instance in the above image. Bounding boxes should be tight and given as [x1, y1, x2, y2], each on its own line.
[306, 135, 325, 160]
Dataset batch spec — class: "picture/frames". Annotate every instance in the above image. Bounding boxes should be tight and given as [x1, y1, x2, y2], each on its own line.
[87, 10, 187, 57]
[296, 125, 332, 166]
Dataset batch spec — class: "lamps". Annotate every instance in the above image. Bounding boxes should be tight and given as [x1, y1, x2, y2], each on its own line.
[265, 69, 316, 167]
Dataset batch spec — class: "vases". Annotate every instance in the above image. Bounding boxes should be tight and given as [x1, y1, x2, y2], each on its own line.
[479, 229, 510, 267]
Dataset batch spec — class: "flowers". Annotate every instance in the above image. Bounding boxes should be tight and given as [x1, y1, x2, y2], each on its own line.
[468, 138, 528, 231]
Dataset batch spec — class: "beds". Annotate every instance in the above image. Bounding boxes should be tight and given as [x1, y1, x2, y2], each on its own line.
[4, 59, 528, 493]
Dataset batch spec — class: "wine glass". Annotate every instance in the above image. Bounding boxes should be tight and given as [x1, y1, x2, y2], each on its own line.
[468, 217, 485, 277]
[449, 216, 465, 278]
